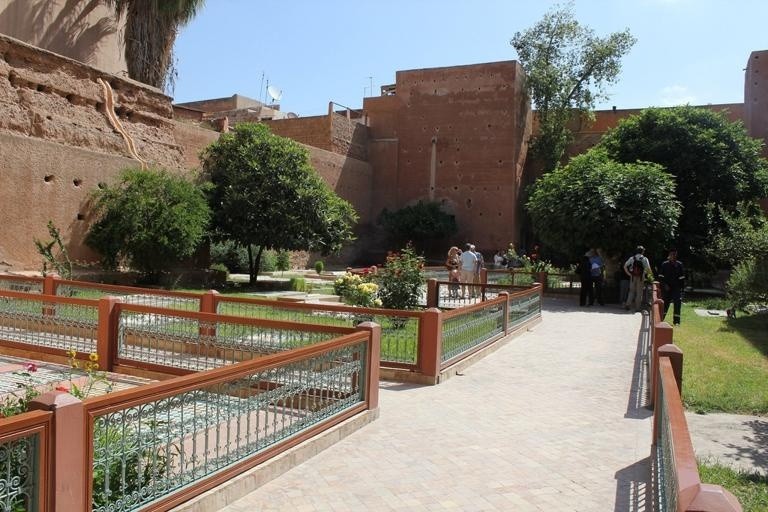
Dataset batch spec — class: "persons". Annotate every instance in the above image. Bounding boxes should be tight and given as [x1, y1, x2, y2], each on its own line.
[624, 246, 652, 312]
[574, 251, 594, 306]
[445, 243, 484, 299]
[590, 249, 605, 306]
[658, 248, 685, 326]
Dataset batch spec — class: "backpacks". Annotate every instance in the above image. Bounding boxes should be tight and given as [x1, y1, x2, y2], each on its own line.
[631, 255, 644, 282]
